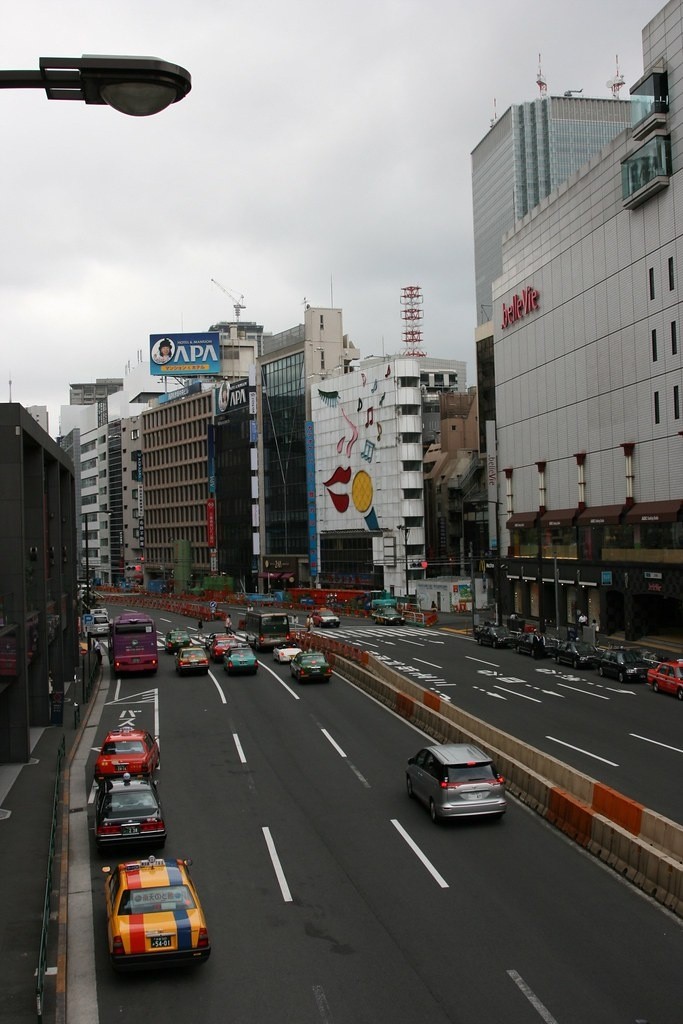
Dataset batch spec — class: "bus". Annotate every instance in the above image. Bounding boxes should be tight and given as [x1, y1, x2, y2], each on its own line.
[245, 610, 291, 650]
[108, 612, 159, 674]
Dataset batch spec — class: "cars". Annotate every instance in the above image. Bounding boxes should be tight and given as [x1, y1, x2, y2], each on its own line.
[205, 632, 239, 662]
[312, 608, 341, 627]
[646, 659, 683, 700]
[594, 649, 650, 683]
[272, 642, 302, 663]
[371, 607, 405, 626]
[96, 727, 161, 776]
[164, 630, 191, 654]
[93, 774, 168, 852]
[554, 641, 602, 669]
[289, 650, 332, 684]
[472, 622, 557, 657]
[222, 647, 258, 675]
[174, 646, 210, 676]
[101, 855, 211, 975]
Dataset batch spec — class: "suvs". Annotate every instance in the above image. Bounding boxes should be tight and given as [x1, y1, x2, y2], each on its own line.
[91, 613, 111, 635]
[404, 743, 507, 824]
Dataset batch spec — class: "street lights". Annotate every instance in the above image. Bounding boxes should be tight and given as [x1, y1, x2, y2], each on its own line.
[82, 510, 114, 610]
[396, 524, 410, 595]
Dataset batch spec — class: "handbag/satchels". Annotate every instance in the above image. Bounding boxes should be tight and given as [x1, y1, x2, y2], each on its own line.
[595, 626, 599, 631]
[305, 623, 310, 627]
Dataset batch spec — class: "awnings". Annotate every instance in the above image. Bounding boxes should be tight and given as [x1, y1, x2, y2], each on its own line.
[258, 572, 281, 579]
[134, 573, 143, 579]
[281, 572, 296, 580]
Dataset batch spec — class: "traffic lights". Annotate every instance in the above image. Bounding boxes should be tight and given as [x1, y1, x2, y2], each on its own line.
[125, 565, 141, 571]
[411, 562, 427, 568]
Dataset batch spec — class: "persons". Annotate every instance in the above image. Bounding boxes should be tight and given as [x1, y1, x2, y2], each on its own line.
[306, 609, 322, 634]
[246, 604, 254, 612]
[539, 616, 548, 635]
[569, 638, 581, 670]
[431, 601, 438, 613]
[590, 620, 599, 644]
[509, 611, 518, 619]
[94, 637, 103, 665]
[567, 628, 579, 642]
[533, 631, 547, 660]
[579, 613, 588, 632]
[48, 673, 54, 703]
[197, 616, 205, 640]
[154, 338, 173, 362]
[225, 613, 236, 635]
